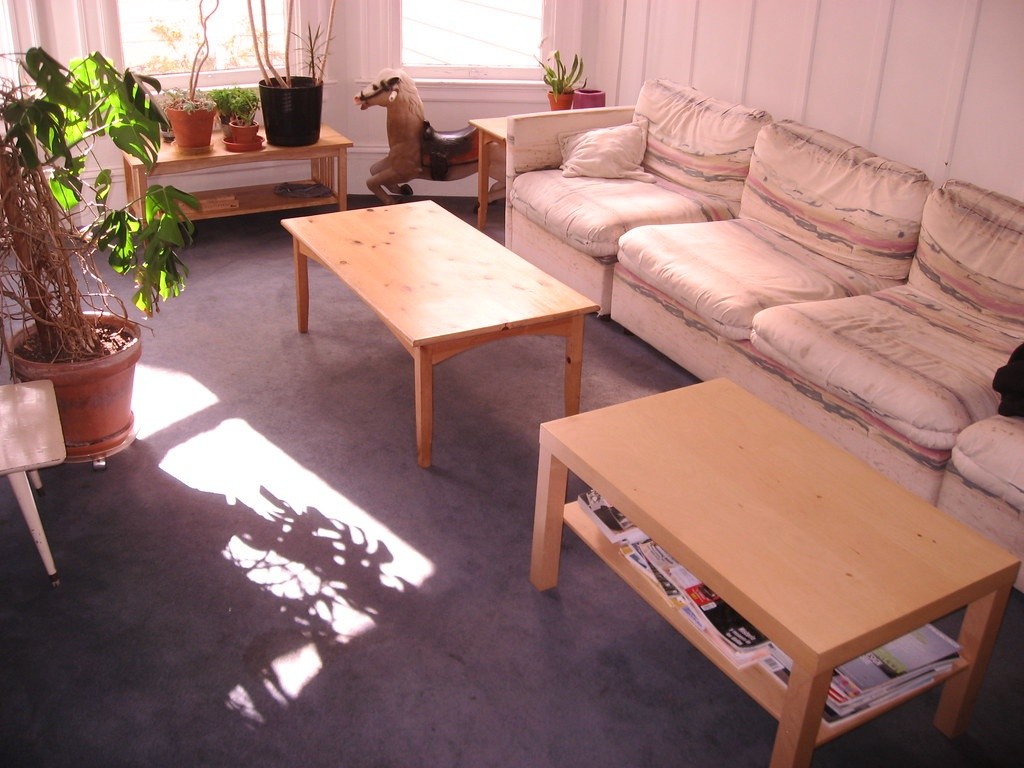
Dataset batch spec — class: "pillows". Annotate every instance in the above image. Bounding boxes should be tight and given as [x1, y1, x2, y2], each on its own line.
[735, 123, 929, 297]
[909, 189, 1024, 324]
[879, 202, 890, 204]
[561, 122, 650, 179]
[631, 76, 771, 217]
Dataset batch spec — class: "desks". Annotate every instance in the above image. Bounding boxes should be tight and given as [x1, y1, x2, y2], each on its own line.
[123, 121, 354, 255]
[0, 379, 66, 589]
[468, 114, 507, 232]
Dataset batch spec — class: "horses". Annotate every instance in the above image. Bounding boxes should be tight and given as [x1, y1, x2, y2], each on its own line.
[354, 67, 507, 212]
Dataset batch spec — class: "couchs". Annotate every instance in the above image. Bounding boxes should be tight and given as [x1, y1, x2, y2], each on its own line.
[501, 109, 1023, 601]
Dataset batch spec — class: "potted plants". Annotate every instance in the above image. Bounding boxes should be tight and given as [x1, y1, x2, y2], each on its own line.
[246, 0, 336, 147]
[0, 45, 202, 465]
[159, 0, 218, 152]
[207, 87, 261, 143]
[537, 52, 587, 112]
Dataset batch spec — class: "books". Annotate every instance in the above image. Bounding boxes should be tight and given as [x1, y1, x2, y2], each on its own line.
[577, 489, 962, 727]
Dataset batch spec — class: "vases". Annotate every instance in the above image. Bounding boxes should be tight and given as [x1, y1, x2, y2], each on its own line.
[576, 89, 605, 106]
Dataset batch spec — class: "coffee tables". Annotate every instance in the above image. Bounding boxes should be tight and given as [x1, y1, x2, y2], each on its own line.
[528, 376, 1024, 768]
[280, 198, 599, 468]
